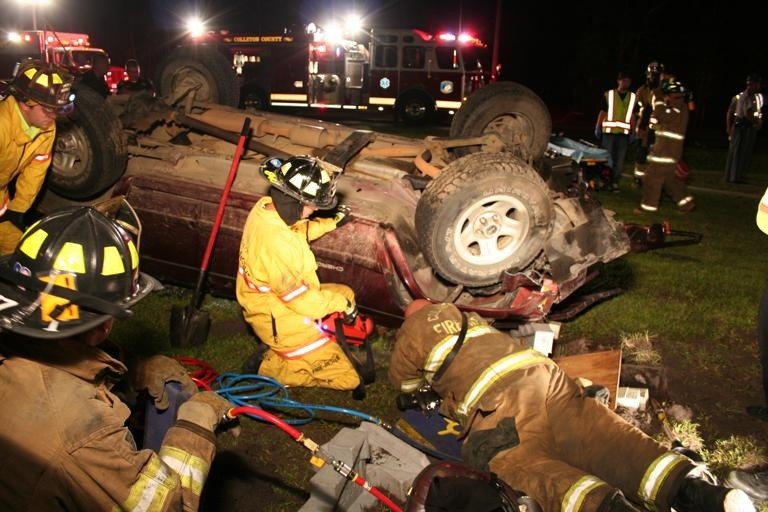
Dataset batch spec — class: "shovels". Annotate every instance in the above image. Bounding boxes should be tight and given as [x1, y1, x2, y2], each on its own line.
[170, 117, 251, 348]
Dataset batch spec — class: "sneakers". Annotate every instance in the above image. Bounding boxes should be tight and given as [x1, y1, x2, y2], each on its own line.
[245, 342, 268, 373]
[633, 201, 696, 214]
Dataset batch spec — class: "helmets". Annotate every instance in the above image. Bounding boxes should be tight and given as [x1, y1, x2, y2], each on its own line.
[745, 74, 762, 82]
[405, 299, 431, 315]
[0, 61, 80, 114]
[258, 154, 339, 211]
[647, 61, 664, 77]
[0, 205, 164, 341]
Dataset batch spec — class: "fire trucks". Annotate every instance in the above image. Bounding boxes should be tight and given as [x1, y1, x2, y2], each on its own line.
[0, 25, 152, 100]
[155, 5, 492, 128]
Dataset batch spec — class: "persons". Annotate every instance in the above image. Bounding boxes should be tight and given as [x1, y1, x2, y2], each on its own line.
[625, 59, 668, 190]
[720, 465, 768, 506]
[1, 57, 68, 255]
[722, 74, 767, 186]
[71, 52, 113, 103]
[745, 184, 768, 425]
[232, 154, 365, 390]
[116, 58, 155, 96]
[386, 298, 756, 511]
[629, 61, 699, 216]
[0, 197, 243, 512]
[236, 60, 272, 112]
[592, 71, 638, 196]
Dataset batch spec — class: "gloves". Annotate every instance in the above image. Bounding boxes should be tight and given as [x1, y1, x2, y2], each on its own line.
[346, 306, 358, 324]
[130, 354, 243, 437]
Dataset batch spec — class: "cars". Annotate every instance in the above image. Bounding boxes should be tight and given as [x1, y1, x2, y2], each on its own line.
[11, 50, 626, 342]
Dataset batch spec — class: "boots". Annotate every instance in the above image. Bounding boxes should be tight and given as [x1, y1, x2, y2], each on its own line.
[611, 470, 768, 512]
